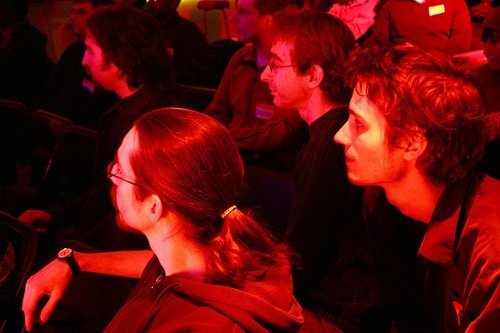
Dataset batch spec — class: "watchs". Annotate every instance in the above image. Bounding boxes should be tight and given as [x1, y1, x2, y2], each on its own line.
[58, 248, 80, 278]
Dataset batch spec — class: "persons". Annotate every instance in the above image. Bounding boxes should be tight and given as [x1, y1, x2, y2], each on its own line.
[333, 43, 500, 333]
[21, 108, 304, 333]
[0, 0, 500, 333]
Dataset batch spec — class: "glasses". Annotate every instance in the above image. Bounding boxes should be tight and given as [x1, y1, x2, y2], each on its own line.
[107, 160, 142, 187]
[269, 58, 296, 72]
